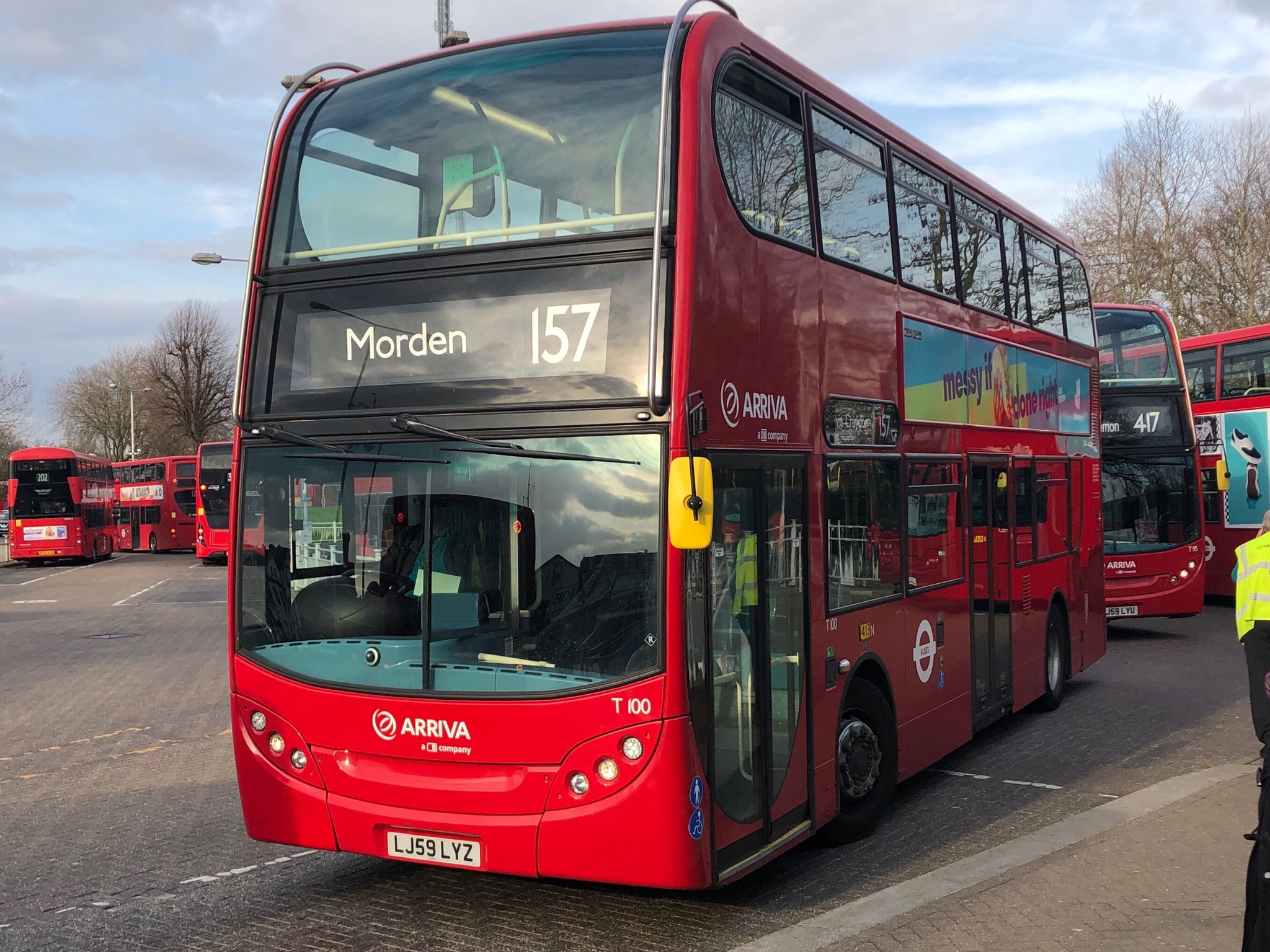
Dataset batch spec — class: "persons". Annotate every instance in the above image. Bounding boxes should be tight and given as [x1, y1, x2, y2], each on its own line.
[378, 486, 487, 637]
[1228, 510, 1270, 951]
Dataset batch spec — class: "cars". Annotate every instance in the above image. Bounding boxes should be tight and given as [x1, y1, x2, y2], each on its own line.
[0, 510, 9, 538]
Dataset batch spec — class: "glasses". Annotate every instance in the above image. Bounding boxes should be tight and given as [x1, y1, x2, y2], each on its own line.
[413, 498, 426, 507]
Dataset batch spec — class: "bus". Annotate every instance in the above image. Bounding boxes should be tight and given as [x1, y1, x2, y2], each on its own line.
[227, 1, 1111, 888]
[1097, 324, 1269, 598]
[1089, 298, 1231, 621]
[110, 456, 198, 555]
[195, 440, 267, 567]
[8, 447, 117, 567]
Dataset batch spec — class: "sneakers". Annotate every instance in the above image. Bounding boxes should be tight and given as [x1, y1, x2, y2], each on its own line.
[1230, 428, 1263, 464]
[1246, 461, 1260, 499]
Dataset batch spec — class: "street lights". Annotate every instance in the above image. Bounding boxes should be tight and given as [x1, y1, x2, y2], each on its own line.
[109, 383, 154, 481]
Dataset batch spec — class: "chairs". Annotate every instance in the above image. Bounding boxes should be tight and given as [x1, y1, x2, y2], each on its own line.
[455, 503, 493, 592]
[1190, 385, 1270, 401]
[1102, 373, 1136, 381]
